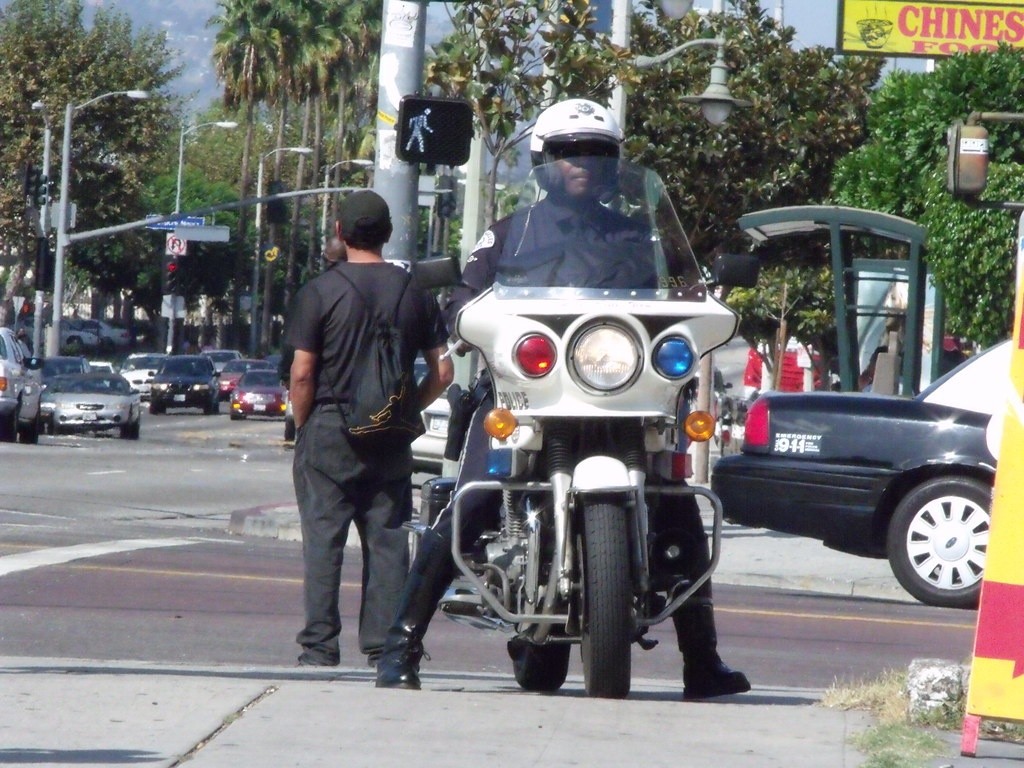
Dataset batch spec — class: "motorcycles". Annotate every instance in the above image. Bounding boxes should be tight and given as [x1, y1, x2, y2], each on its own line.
[396, 155, 747, 699]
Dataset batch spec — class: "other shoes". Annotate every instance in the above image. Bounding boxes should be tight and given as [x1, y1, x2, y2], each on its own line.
[298, 650, 333, 666]
[282, 441, 296, 449]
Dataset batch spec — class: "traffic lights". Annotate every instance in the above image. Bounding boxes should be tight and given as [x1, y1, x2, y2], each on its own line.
[22, 161, 48, 206]
[164, 260, 177, 294]
[395, 96, 474, 167]
[20, 302, 36, 317]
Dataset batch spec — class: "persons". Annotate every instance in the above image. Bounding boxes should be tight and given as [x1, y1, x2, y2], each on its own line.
[376, 97, 753, 699]
[283, 191, 454, 668]
[715, 408, 735, 448]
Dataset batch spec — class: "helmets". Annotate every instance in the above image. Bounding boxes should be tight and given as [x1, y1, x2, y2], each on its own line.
[530, 99, 622, 203]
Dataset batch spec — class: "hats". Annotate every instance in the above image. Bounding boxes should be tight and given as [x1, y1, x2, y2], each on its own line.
[340, 190, 391, 238]
[323, 236, 348, 263]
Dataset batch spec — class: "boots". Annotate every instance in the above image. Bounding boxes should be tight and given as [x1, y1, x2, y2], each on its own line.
[377, 528, 461, 690]
[663, 532, 752, 698]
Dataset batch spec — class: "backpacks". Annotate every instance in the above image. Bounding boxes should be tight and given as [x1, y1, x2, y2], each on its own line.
[320, 269, 426, 450]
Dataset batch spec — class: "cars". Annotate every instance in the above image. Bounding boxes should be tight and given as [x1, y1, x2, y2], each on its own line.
[0, 317, 296, 443]
[402, 354, 454, 475]
[709, 332, 1012, 609]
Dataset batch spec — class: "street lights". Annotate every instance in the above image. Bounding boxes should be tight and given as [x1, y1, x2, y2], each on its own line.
[593, 31, 754, 212]
[249, 147, 313, 358]
[32, 99, 52, 361]
[319, 158, 376, 275]
[48, 90, 153, 356]
[166, 121, 239, 356]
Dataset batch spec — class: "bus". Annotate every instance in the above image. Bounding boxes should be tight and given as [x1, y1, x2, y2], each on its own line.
[943, 108, 1024, 761]
